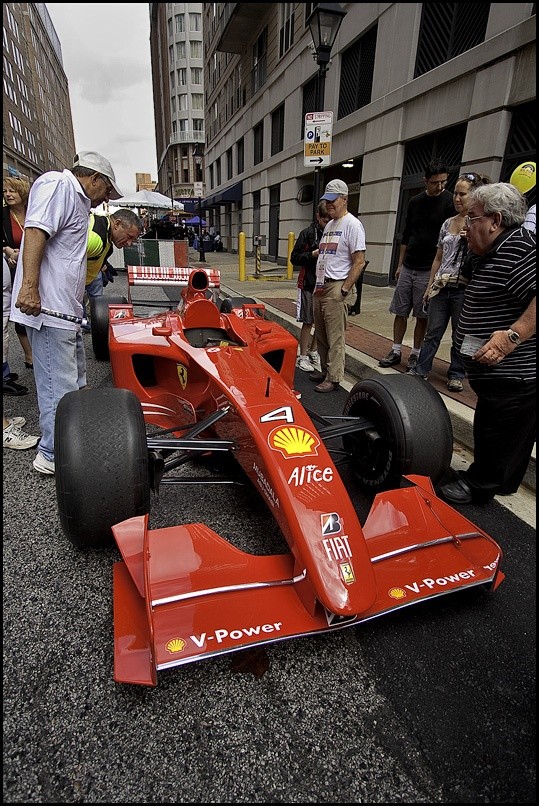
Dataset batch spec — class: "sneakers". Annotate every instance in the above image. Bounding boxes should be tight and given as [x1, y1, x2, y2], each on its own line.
[6, 417, 26, 429]
[33, 452, 55, 474]
[378, 349, 402, 367]
[308, 353, 322, 372]
[406, 354, 419, 375]
[3, 423, 41, 450]
[447, 378, 464, 391]
[440, 479, 474, 502]
[416, 370, 428, 381]
[295, 355, 314, 372]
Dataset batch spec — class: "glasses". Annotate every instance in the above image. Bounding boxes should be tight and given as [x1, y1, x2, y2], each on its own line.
[458, 175, 476, 184]
[99, 176, 112, 198]
[464, 212, 506, 227]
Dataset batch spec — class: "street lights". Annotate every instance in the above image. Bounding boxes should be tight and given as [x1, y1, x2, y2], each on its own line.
[307, 0, 348, 223]
[166, 165, 174, 216]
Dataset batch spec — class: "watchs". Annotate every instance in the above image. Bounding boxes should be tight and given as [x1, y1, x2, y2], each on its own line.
[341, 289, 348, 297]
[506, 329, 521, 345]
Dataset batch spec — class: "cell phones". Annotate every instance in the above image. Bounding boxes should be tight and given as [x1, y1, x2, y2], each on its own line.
[459, 335, 487, 357]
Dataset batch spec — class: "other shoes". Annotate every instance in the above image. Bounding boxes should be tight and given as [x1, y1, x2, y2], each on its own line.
[3, 372, 19, 381]
[1, 380, 29, 395]
[24, 361, 34, 369]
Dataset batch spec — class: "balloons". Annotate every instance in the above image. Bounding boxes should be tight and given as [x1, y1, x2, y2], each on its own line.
[509, 161, 536, 194]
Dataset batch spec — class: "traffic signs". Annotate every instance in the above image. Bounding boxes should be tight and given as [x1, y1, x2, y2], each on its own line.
[304, 112, 334, 169]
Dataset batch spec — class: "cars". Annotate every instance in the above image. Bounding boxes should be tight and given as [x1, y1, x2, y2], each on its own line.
[138, 225, 196, 244]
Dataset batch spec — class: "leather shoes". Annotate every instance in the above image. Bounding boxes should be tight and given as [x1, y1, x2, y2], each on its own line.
[315, 380, 339, 392]
[309, 371, 327, 381]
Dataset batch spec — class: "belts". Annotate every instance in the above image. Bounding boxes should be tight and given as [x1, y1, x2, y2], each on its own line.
[324, 278, 335, 282]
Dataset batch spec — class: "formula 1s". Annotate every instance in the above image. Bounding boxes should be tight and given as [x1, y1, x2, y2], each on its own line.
[53, 271, 505, 687]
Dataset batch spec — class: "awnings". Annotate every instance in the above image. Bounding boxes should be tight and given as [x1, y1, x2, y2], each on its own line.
[194, 181, 242, 211]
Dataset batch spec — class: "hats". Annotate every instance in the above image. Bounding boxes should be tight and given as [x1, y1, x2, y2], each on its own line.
[320, 179, 348, 201]
[72, 152, 124, 200]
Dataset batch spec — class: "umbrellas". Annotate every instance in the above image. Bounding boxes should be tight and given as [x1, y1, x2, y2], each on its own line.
[108, 189, 184, 226]
[184, 216, 206, 226]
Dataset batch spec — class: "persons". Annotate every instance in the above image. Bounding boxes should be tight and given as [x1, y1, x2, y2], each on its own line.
[2, 152, 144, 475]
[204, 231, 221, 252]
[308, 179, 365, 392]
[439, 182, 536, 504]
[290, 200, 332, 372]
[376, 158, 536, 392]
